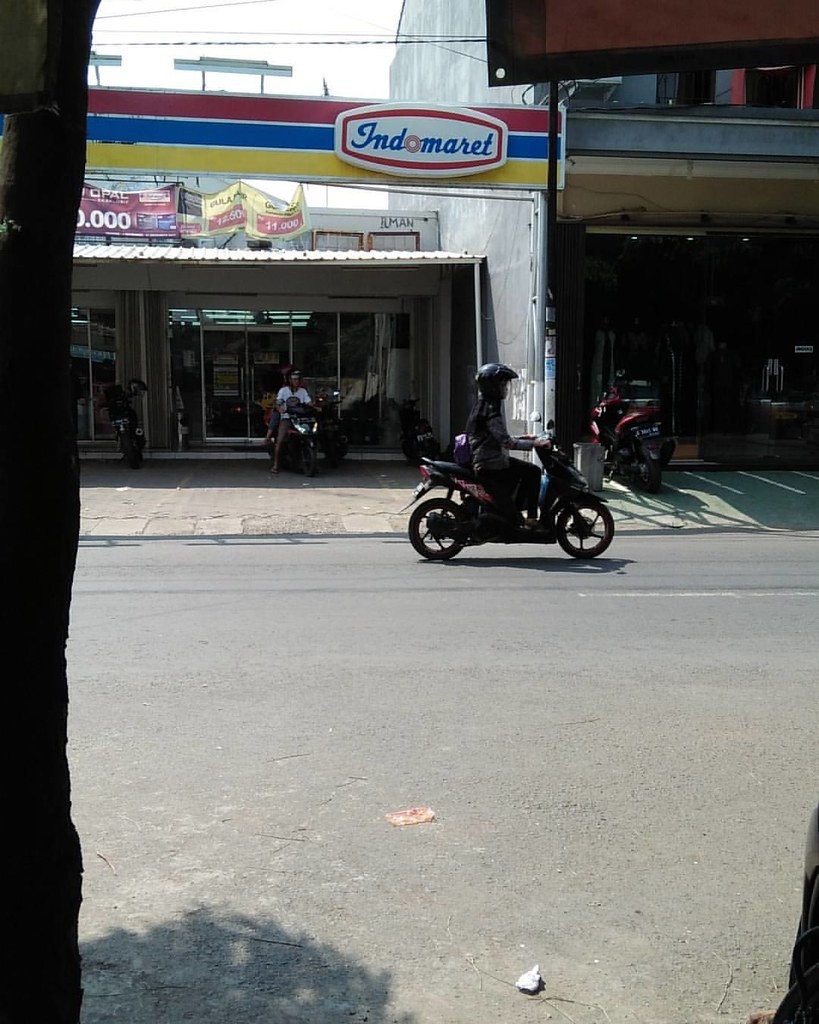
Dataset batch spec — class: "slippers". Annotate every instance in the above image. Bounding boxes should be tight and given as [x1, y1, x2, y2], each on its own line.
[271, 466, 279, 474]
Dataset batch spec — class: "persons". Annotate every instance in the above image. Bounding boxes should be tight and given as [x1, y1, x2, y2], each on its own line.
[465, 363, 551, 530]
[262, 364, 315, 473]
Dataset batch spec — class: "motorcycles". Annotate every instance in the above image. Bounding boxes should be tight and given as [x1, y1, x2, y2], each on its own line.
[99, 383, 147, 470]
[591, 368, 676, 493]
[396, 397, 441, 468]
[399, 410, 615, 560]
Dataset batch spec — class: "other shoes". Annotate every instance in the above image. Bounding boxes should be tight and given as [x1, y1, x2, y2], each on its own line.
[523, 520, 551, 533]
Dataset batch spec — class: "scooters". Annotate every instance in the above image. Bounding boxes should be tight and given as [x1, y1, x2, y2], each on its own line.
[268, 390, 349, 476]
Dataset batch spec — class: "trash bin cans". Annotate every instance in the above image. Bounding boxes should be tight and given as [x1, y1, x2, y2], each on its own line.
[573, 442, 606, 491]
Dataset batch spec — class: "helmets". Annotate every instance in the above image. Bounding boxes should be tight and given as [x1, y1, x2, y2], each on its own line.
[279, 367, 291, 374]
[285, 368, 303, 383]
[128, 379, 148, 398]
[474, 363, 518, 386]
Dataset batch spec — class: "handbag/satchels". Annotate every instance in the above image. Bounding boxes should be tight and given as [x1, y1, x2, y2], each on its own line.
[454, 433, 471, 467]
